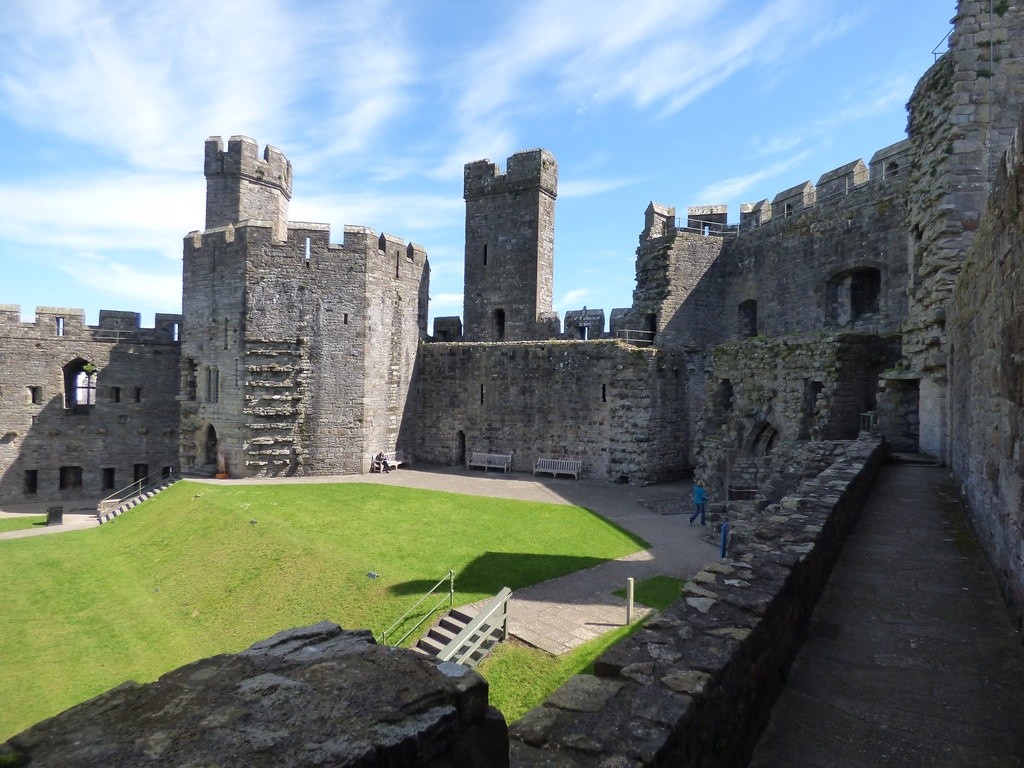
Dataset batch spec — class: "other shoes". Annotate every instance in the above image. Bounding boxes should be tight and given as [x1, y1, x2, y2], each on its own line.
[688, 519, 692, 525]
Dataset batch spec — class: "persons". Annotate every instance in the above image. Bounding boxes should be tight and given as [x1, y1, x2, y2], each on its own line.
[688, 480, 708, 526]
[375, 450, 392, 473]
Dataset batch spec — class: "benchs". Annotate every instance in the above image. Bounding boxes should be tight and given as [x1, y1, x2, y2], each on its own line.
[532, 456, 583, 481]
[372, 448, 412, 474]
[466, 451, 512, 475]
